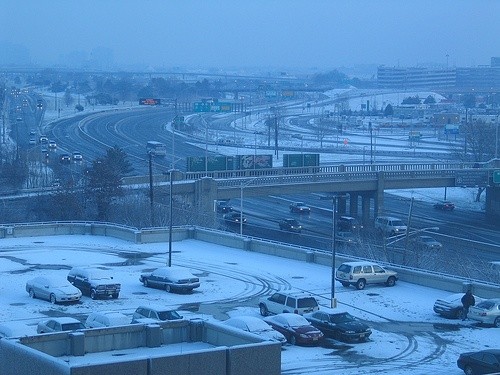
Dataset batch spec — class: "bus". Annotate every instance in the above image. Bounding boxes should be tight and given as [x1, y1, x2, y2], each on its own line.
[147, 141, 167, 158]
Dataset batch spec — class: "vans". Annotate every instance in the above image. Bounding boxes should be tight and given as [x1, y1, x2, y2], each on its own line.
[375, 216, 407, 237]
[131, 305, 184, 324]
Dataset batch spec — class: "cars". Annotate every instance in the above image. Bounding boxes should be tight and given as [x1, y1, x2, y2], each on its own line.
[139, 266, 201, 293]
[37, 317, 85, 336]
[85, 310, 130, 329]
[217, 201, 247, 225]
[289, 201, 311, 214]
[222, 315, 287, 347]
[264, 313, 324, 346]
[26, 273, 83, 304]
[456, 349, 500, 375]
[303, 308, 372, 342]
[434, 199, 455, 211]
[11, 87, 83, 165]
[433, 293, 487, 320]
[466, 297, 500, 328]
[414, 236, 442, 250]
[279, 218, 303, 233]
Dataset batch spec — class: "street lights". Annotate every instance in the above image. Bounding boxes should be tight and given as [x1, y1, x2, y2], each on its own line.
[383, 227, 440, 262]
[331, 195, 350, 309]
[167, 168, 180, 267]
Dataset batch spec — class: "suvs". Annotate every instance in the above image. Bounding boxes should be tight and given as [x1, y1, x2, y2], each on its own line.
[335, 216, 364, 246]
[67, 266, 121, 300]
[335, 261, 399, 290]
[257, 290, 320, 319]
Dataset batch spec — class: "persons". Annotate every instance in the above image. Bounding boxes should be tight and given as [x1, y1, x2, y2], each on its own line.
[461, 289, 475, 321]
